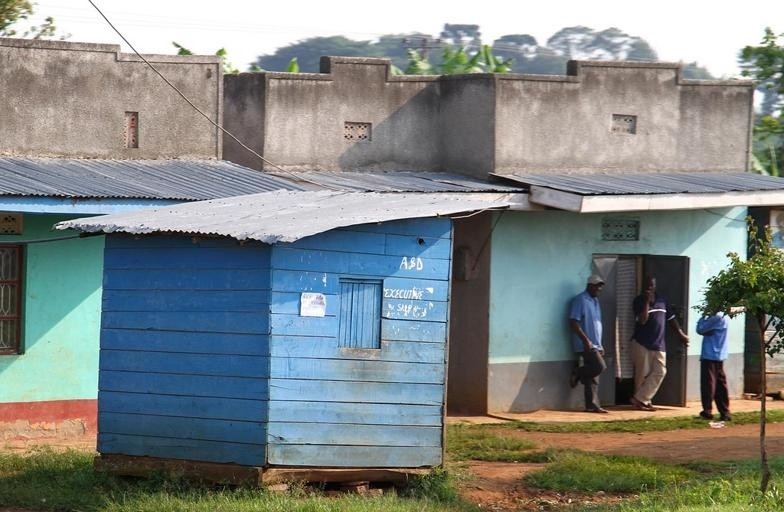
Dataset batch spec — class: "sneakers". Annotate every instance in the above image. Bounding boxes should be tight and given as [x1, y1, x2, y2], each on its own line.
[700, 411, 732, 420]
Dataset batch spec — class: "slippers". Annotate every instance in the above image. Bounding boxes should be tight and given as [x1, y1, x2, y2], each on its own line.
[631, 398, 656, 411]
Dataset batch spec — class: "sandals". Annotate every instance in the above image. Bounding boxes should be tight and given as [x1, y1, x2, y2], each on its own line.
[570, 365, 579, 387]
[585, 408, 608, 413]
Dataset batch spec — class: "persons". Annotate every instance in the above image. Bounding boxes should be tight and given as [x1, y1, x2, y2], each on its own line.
[569, 275, 608, 413]
[629, 273, 689, 410]
[696, 311, 731, 421]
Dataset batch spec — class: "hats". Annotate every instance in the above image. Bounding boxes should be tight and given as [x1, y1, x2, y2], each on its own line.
[586, 275, 605, 285]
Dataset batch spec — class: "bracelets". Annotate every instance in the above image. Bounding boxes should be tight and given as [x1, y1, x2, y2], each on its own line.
[582, 336, 588, 343]
